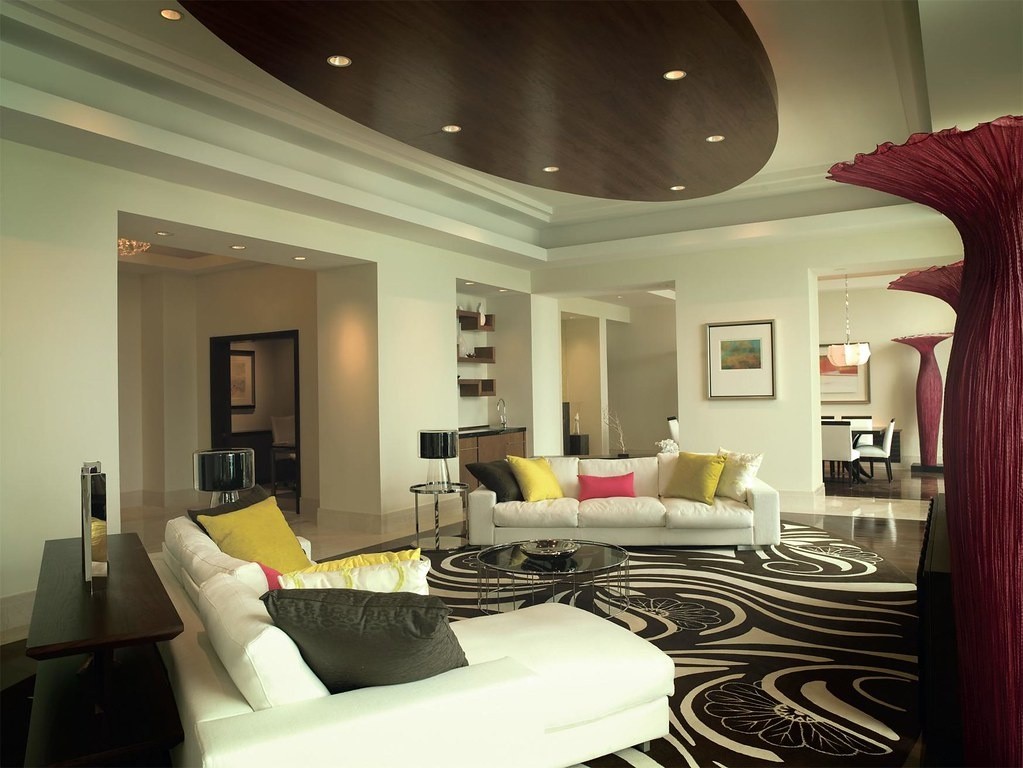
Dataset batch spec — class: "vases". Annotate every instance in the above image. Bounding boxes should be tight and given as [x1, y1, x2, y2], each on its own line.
[888, 333, 956, 466]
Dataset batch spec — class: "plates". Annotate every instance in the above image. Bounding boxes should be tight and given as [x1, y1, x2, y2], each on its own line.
[520, 539, 581, 560]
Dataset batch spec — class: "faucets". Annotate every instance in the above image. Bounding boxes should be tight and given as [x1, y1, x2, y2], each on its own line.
[497, 398, 507, 427]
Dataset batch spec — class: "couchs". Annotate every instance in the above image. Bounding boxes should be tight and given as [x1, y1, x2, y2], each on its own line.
[467, 455, 780, 551]
[144, 516, 674, 768]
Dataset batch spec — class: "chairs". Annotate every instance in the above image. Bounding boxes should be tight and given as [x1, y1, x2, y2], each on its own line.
[821, 416, 895, 484]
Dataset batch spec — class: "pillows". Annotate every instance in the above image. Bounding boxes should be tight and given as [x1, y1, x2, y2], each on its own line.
[715, 447, 762, 502]
[187, 485, 467, 693]
[465, 460, 524, 502]
[664, 451, 728, 505]
[506, 455, 564, 501]
[576, 472, 635, 502]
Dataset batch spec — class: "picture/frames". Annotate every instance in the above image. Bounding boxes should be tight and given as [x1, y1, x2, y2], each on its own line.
[230, 349, 257, 407]
[704, 319, 776, 400]
[820, 343, 871, 405]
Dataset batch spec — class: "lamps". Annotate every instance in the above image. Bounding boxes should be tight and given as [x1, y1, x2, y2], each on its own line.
[418, 429, 460, 489]
[826, 274, 871, 367]
[192, 449, 255, 508]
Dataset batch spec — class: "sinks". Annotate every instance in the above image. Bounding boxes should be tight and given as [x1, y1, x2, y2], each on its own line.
[458, 429, 490, 434]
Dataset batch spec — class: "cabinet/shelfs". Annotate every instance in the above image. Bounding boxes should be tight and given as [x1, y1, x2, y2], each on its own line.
[458, 310, 498, 396]
[459, 432, 526, 506]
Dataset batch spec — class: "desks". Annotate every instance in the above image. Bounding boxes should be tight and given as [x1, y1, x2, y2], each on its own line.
[410, 482, 470, 550]
[23, 531, 185, 746]
[851, 425, 887, 483]
